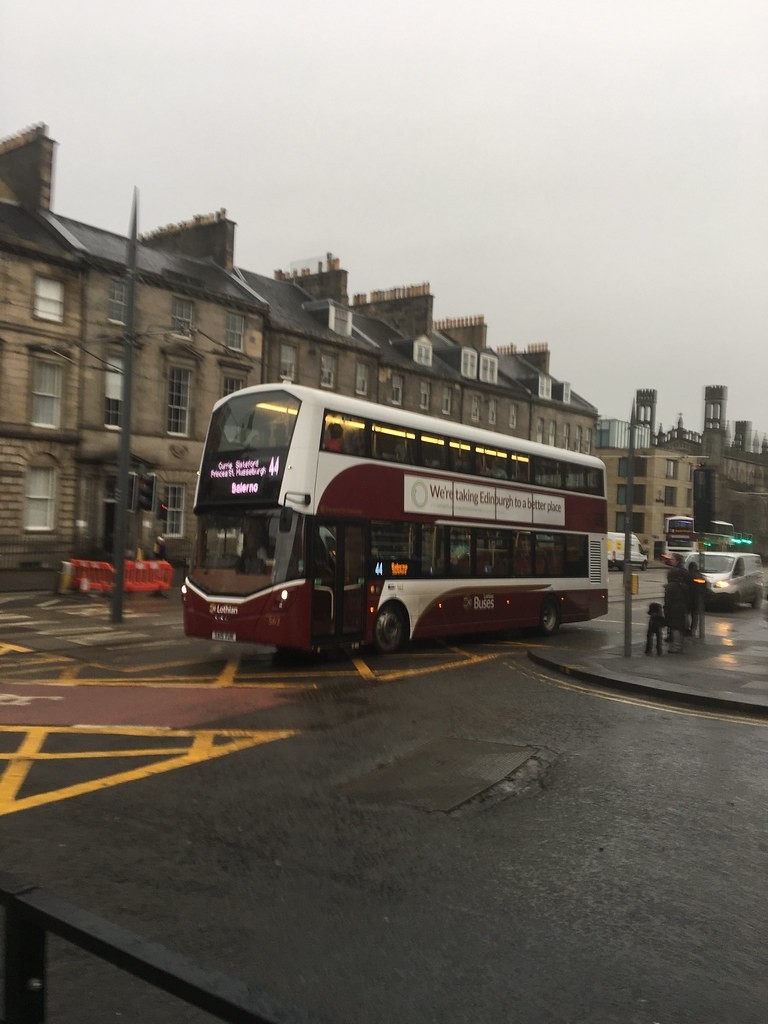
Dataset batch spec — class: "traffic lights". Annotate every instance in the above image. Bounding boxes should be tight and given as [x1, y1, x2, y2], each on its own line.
[137, 472, 155, 511]
[734, 531, 753, 545]
[158, 497, 169, 520]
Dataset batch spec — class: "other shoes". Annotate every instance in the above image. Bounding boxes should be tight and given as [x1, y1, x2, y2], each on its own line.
[668, 645, 683, 653]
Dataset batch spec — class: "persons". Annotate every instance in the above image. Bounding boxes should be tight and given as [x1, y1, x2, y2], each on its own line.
[662, 553, 704, 654]
[324, 422, 344, 450]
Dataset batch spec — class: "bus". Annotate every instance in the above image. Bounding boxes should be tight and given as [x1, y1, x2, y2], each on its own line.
[180, 380, 610, 657]
[660, 515, 734, 563]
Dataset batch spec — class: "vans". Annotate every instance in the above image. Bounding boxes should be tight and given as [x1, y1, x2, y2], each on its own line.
[606, 531, 647, 571]
[683, 551, 764, 609]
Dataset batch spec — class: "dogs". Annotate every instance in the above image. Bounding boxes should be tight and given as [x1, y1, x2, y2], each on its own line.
[644, 601, 668, 656]
